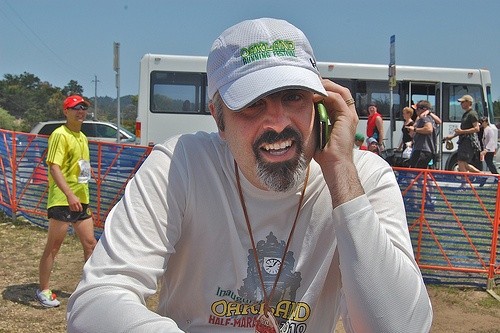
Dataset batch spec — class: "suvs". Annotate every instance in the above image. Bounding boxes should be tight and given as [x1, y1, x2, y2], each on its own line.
[23, 119, 137, 167]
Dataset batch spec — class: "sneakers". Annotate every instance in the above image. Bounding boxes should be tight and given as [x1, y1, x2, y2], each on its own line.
[34, 289, 60, 308]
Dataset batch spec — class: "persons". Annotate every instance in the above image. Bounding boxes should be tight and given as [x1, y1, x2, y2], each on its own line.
[366, 136, 379, 154]
[365, 101, 386, 150]
[443, 95, 488, 192]
[69, 16, 432, 333]
[398, 107, 415, 167]
[478, 116, 499, 187]
[353, 131, 368, 150]
[404, 99, 442, 212]
[36, 95, 97, 307]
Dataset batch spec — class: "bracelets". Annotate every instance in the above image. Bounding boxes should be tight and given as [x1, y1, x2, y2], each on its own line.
[428, 110, 431, 115]
[414, 126, 416, 132]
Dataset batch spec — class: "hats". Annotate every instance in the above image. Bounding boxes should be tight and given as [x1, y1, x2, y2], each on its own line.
[412, 104, 417, 110]
[367, 137, 378, 145]
[457, 95, 473, 102]
[64, 95, 91, 110]
[206, 18, 328, 111]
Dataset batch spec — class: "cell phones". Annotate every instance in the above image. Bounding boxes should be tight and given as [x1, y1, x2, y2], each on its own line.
[315, 99, 329, 150]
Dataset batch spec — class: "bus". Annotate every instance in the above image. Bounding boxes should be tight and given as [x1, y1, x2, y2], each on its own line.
[133, 53, 497, 185]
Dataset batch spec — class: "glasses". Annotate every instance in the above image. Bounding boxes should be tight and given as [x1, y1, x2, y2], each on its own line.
[72, 104, 88, 110]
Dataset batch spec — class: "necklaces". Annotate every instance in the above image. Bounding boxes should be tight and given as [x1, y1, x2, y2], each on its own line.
[232, 160, 309, 333]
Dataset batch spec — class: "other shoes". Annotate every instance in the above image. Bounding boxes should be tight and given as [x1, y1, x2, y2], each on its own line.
[480, 177, 488, 187]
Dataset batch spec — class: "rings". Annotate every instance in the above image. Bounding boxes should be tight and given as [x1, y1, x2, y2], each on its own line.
[344, 98, 354, 105]
